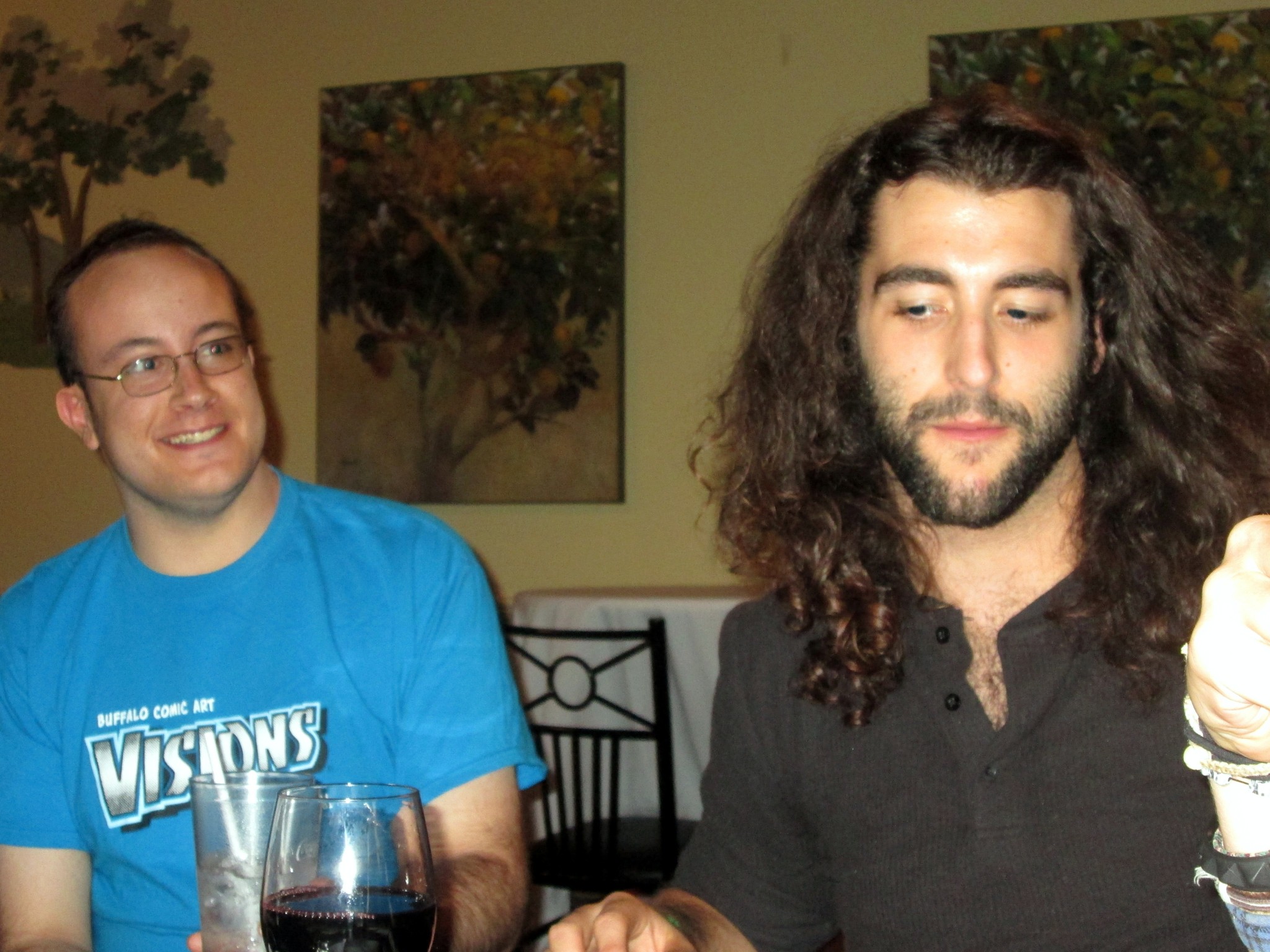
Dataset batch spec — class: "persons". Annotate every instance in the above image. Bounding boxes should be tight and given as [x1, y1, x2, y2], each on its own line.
[547, 83, 1258, 952]
[1179, 514, 1270, 952]
[0, 218, 548, 952]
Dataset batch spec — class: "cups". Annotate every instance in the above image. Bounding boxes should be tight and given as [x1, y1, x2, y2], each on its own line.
[190, 771, 320, 951]
[259, 782, 440, 952]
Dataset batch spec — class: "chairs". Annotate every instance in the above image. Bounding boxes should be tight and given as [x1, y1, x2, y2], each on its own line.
[484, 608, 700, 905]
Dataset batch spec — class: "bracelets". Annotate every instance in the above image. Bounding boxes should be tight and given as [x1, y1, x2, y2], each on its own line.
[1182, 695, 1270, 799]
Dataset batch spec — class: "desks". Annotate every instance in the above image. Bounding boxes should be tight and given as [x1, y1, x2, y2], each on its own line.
[483, 582, 763, 936]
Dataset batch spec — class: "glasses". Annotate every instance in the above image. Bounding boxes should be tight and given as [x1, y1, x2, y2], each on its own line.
[77, 334, 256, 398]
